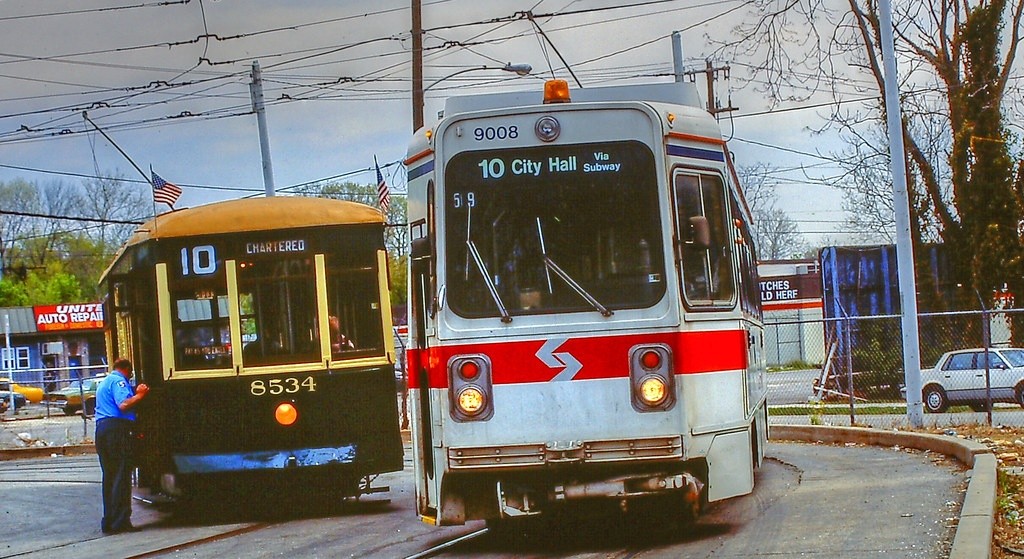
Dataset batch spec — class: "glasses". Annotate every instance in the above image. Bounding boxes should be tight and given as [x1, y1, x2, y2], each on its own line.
[127, 369, 133, 378]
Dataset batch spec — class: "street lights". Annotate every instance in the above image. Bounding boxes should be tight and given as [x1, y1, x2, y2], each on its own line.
[413, 62, 534, 133]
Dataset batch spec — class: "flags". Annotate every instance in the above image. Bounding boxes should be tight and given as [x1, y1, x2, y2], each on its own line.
[149, 171, 181, 208]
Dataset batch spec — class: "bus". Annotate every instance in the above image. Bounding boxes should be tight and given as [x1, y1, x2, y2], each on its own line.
[96, 195, 406, 518]
[412, 78, 769, 550]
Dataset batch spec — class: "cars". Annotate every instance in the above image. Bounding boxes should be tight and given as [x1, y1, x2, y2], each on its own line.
[0, 377, 45, 415]
[40, 377, 105, 417]
[899, 347, 1024, 413]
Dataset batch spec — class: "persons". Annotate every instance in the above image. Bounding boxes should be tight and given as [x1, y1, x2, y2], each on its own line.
[94, 359, 150, 534]
[244, 315, 353, 360]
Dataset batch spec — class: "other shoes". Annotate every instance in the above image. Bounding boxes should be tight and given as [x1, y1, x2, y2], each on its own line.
[102, 526, 141, 534]
